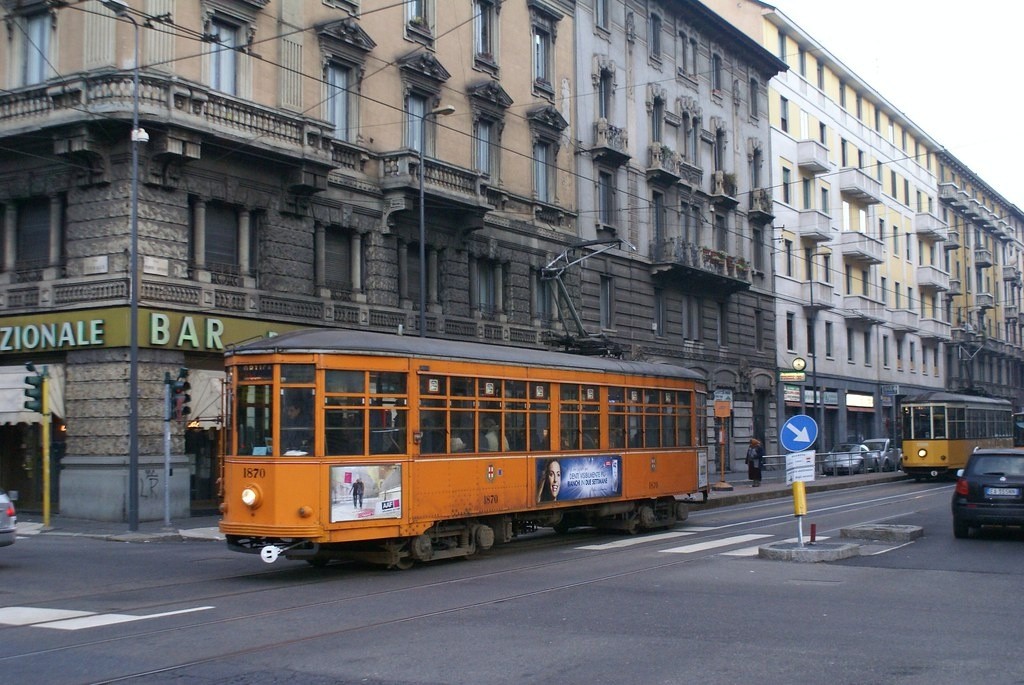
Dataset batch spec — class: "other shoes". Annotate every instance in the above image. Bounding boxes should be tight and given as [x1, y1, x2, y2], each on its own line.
[752, 482, 759, 486]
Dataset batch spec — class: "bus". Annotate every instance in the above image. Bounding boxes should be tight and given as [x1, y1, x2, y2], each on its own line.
[901, 392, 1015, 481]
[217, 328, 711, 568]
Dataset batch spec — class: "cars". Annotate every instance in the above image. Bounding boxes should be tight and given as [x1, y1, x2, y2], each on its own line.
[863, 438, 900, 471]
[0, 488, 17, 548]
[822, 443, 870, 474]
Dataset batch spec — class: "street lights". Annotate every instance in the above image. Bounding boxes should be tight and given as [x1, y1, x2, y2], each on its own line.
[420, 105, 456, 337]
[102, 0, 140, 531]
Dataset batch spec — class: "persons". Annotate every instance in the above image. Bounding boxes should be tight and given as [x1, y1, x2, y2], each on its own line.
[422, 400, 550, 453]
[281, 394, 314, 449]
[610, 423, 632, 448]
[326, 398, 406, 455]
[745, 439, 763, 487]
[574, 420, 600, 449]
[349, 477, 364, 508]
[538, 458, 562, 502]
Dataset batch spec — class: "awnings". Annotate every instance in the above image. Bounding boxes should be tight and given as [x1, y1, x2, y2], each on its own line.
[0, 364, 65, 425]
[186, 370, 272, 430]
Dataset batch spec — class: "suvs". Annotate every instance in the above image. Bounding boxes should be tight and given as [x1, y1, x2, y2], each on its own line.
[951, 446, 1024, 538]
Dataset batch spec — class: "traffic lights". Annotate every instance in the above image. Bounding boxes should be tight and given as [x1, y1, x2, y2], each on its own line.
[24, 377, 42, 411]
[170, 381, 192, 417]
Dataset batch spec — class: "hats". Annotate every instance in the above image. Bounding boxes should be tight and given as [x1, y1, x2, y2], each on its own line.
[750, 439, 761, 446]
